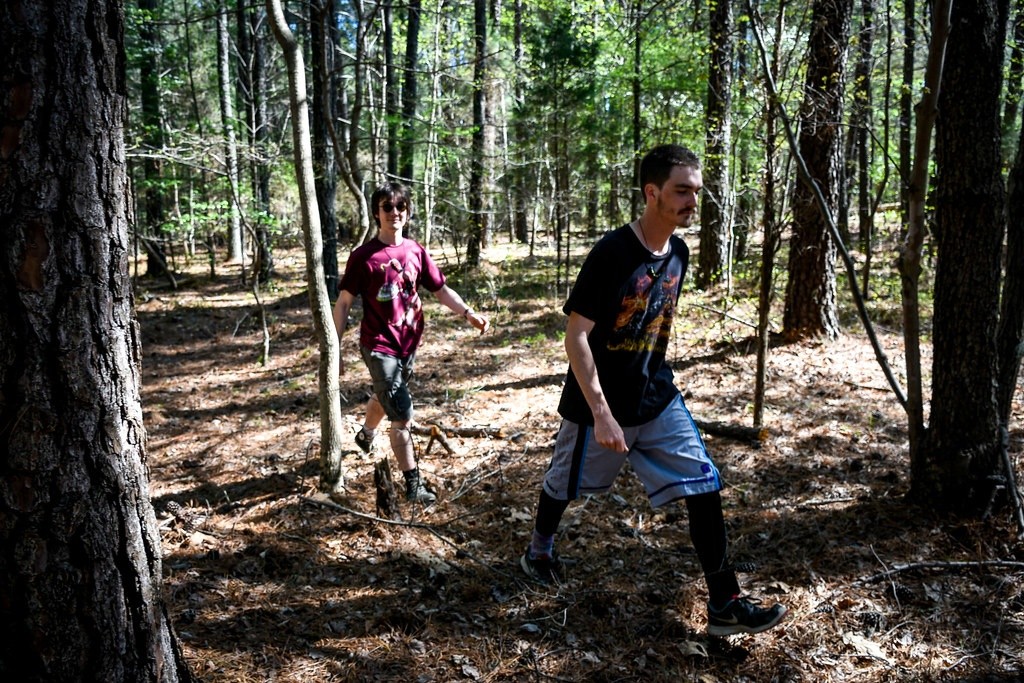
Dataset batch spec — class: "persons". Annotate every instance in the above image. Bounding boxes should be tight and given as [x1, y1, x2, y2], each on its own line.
[332, 182, 490, 508]
[522, 145, 790, 637]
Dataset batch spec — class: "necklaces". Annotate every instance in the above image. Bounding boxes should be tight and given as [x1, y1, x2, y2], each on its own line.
[639, 219, 670, 277]
[384, 238, 407, 272]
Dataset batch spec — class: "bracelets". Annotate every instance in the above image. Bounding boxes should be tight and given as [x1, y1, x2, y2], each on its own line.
[464, 309, 475, 320]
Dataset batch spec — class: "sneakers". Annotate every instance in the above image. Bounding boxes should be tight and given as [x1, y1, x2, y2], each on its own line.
[406, 478, 436, 506]
[705, 595, 788, 637]
[353, 430, 377, 456]
[520, 546, 577, 592]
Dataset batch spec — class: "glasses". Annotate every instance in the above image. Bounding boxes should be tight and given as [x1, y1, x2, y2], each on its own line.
[381, 202, 406, 212]
